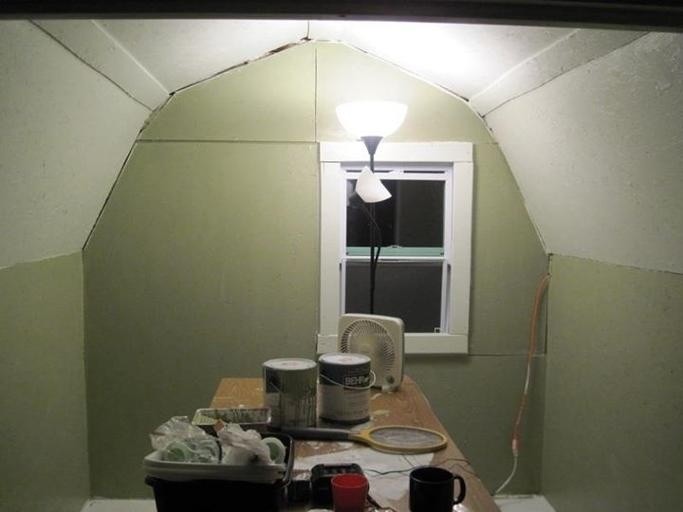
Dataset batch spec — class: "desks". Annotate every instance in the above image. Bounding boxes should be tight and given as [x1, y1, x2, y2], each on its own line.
[210, 375, 502, 512]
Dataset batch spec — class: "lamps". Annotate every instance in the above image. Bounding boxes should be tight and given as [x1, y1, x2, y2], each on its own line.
[335, 99, 407, 315]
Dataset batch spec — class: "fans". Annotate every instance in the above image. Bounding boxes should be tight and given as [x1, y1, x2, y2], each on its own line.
[337, 312, 405, 392]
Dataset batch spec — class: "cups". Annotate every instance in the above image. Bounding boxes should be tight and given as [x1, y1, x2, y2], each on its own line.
[331, 473, 368, 512]
[409, 467, 466, 512]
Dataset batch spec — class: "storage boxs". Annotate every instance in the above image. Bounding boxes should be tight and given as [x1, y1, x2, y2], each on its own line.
[144, 432, 295, 512]
